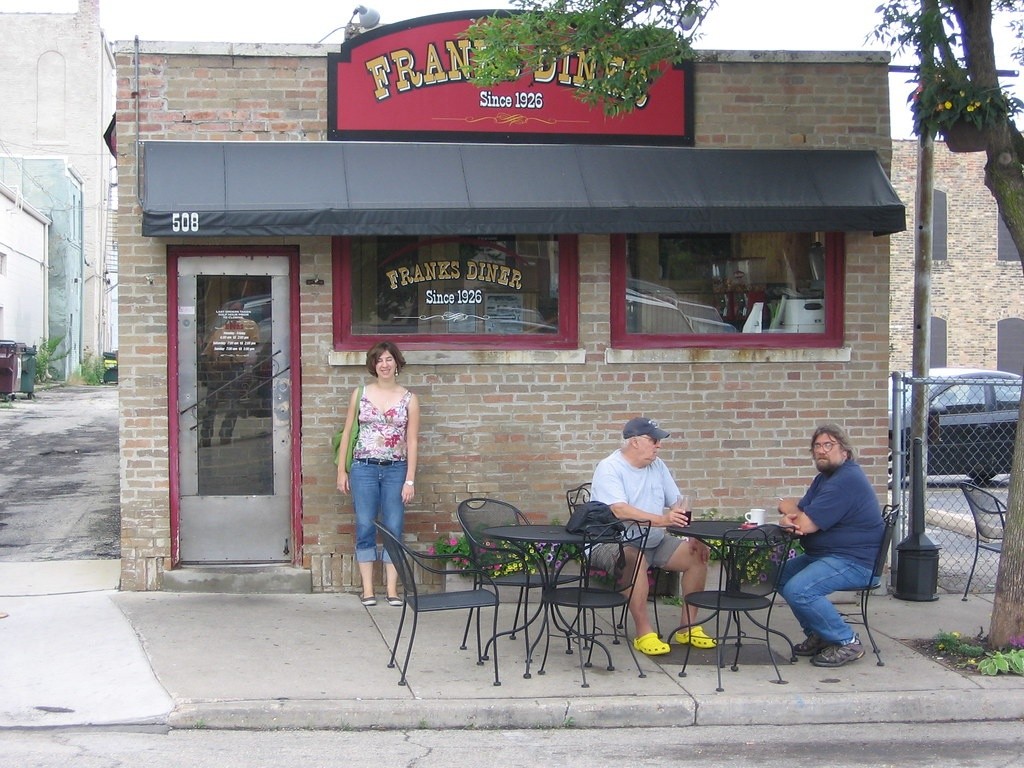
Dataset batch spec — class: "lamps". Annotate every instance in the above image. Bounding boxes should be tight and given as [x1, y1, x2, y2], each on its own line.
[349, 4, 380, 31]
[676, 9, 702, 32]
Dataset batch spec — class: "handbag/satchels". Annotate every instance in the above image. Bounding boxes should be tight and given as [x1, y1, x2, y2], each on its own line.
[329, 385, 359, 472]
[566, 500, 627, 569]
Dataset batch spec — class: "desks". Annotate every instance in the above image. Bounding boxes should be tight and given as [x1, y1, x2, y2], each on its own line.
[666, 520, 803, 671]
[480, 525, 623, 679]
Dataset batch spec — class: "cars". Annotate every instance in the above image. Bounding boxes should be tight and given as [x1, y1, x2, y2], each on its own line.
[889, 368, 1024, 489]
[626, 277, 738, 333]
[201, 293, 272, 414]
[489, 307, 546, 324]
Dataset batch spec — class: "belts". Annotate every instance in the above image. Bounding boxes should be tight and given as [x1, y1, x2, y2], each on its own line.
[357, 458, 399, 465]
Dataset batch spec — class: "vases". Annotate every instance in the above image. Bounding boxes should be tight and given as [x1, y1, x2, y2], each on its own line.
[669, 558, 775, 602]
[446, 532, 636, 603]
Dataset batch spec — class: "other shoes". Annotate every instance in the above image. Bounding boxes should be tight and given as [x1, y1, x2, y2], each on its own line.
[360, 593, 377, 605]
[203, 439, 211, 446]
[385, 595, 403, 606]
[221, 435, 231, 444]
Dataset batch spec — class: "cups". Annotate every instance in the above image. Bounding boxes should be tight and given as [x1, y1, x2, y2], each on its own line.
[677, 495, 692, 527]
[745, 509, 765, 526]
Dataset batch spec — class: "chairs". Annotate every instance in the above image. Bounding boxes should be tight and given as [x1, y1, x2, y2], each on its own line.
[959, 481, 1007, 602]
[834, 504, 900, 666]
[372, 519, 501, 687]
[679, 523, 796, 693]
[456, 497, 591, 666]
[523, 517, 651, 688]
[566, 482, 663, 646]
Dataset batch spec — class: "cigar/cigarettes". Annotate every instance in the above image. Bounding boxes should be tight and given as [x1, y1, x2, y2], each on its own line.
[779, 497, 784, 502]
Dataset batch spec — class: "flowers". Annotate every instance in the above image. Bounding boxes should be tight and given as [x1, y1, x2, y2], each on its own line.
[428, 519, 654, 590]
[677, 508, 805, 587]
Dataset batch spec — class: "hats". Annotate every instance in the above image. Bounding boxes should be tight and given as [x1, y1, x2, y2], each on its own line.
[624, 417, 670, 439]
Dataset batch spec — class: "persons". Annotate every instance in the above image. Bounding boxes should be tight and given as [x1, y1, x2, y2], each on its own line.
[583, 417, 718, 655]
[201, 301, 259, 447]
[723, 271, 749, 318]
[337, 341, 420, 606]
[773, 423, 887, 667]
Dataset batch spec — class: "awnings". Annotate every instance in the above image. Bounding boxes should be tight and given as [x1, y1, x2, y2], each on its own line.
[142, 140, 906, 238]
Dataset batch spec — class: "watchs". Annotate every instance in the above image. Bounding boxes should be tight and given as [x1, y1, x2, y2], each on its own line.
[404, 480, 414, 486]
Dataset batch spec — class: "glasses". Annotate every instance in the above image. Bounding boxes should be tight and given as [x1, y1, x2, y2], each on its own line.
[814, 441, 841, 452]
[637, 435, 660, 444]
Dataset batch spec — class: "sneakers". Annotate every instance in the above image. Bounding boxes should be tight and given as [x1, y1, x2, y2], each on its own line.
[811, 633, 865, 665]
[793, 633, 830, 655]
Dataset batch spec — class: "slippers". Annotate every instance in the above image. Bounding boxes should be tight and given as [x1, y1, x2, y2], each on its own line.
[634, 631, 672, 654]
[675, 625, 717, 648]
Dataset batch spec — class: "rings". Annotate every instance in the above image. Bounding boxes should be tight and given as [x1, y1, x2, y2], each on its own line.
[337, 487, 339, 490]
